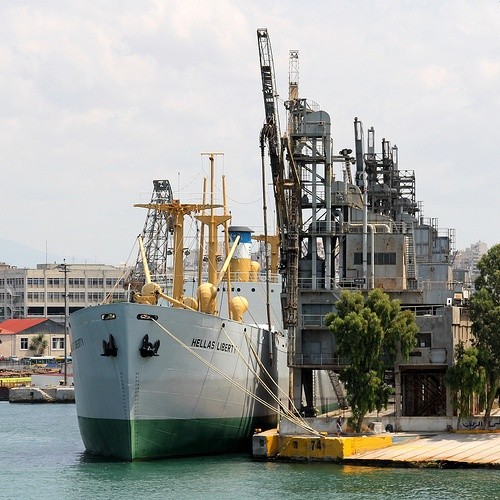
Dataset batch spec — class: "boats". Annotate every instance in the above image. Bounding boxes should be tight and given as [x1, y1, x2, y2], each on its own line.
[8, 372, 76, 403]
[66, 150, 288, 461]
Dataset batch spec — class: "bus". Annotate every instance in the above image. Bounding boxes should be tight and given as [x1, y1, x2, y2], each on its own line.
[21, 356, 73, 372]
